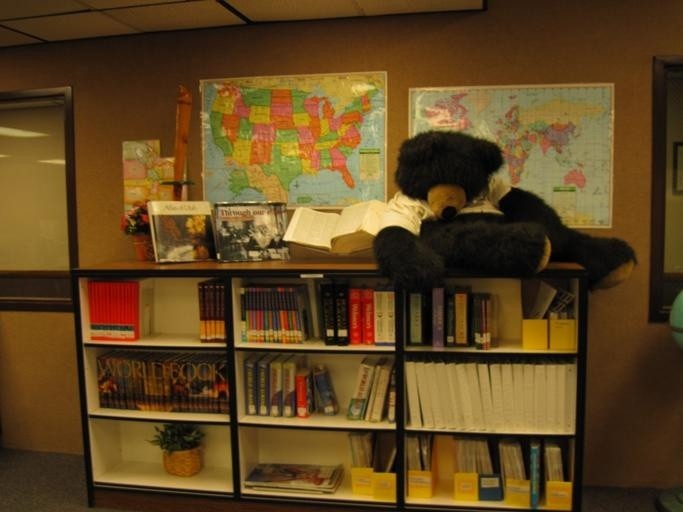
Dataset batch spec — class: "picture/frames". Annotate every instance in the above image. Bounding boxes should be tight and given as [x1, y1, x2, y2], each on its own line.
[673, 142, 683, 195]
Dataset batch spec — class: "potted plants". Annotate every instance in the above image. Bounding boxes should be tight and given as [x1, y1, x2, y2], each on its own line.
[146, 421, 204, 478]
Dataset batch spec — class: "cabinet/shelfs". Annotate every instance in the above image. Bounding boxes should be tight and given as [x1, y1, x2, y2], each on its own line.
[69, 261, 588, 512]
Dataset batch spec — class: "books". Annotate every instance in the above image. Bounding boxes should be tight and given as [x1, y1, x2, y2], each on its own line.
[344, 430, 397, 472]
[210, 200, 292, 263]
[88, 275, 231, 416]
[280, 198, 387, 265]
[145, 200, 219, 265]
[247, 463, 345, 491]
[405, 285, 576, 510]
[239, 280, 395, 422]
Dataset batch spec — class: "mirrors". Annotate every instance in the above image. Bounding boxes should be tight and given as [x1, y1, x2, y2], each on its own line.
[647, 54, 683, 325]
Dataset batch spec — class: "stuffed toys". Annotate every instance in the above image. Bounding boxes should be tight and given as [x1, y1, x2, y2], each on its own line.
[369, 130, 637, 298]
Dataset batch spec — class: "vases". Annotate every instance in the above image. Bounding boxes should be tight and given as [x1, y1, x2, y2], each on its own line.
[132, 234, 155, 262]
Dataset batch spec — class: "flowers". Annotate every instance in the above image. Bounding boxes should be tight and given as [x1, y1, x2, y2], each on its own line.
[119, 197, 152, 235]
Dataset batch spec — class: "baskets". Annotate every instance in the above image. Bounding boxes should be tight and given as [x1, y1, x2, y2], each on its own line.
[164, 446, 204, 476]
